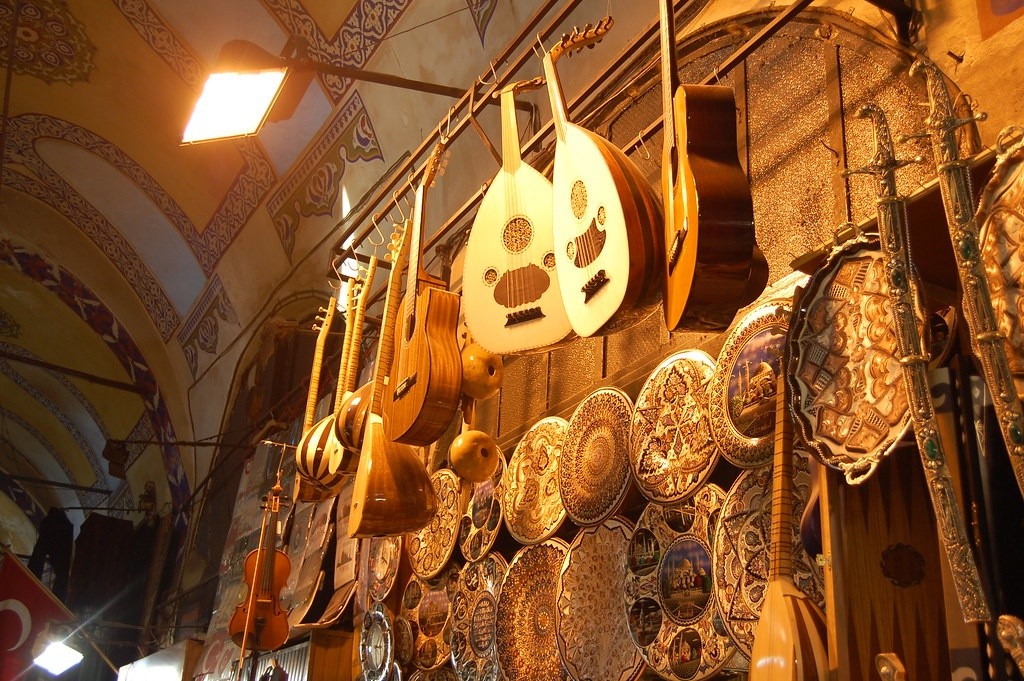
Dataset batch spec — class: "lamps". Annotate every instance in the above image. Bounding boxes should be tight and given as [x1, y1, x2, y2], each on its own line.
[101, 438, 251, 478]
[179, 37, 541, 157]
[32, 634, 163, 677]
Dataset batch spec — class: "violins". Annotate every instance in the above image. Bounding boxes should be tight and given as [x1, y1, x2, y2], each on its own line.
[228, 486, 291, 653]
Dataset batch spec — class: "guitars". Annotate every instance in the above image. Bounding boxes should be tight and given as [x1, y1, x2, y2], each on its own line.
[380, 141, 462, 447]
[660, 1, 753, 333]
